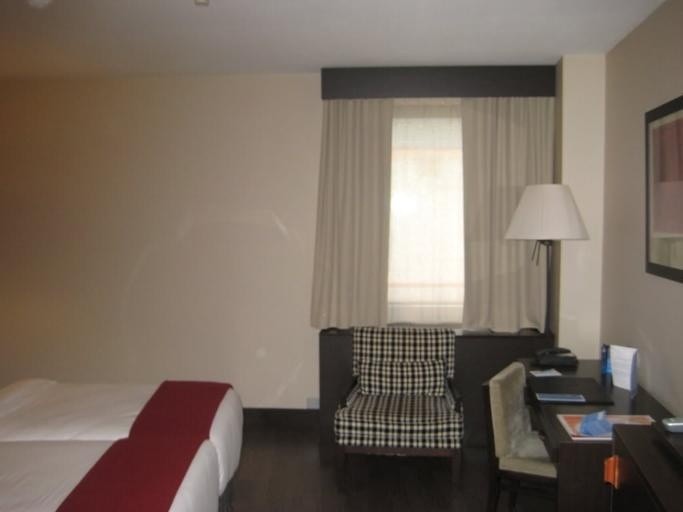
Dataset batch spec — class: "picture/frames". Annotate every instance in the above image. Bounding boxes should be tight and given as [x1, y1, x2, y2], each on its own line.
[503, 183, 592, 333]
[645, 96, 682, 281]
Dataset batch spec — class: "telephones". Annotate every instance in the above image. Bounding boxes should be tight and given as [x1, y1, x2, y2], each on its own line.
[534, 347, 578, 366]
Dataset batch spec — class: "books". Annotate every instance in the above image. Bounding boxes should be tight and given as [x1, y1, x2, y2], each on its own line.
[556, 413, 656, 441]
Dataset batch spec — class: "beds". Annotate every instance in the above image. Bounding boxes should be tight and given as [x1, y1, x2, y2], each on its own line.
[1, 380, 244, 510]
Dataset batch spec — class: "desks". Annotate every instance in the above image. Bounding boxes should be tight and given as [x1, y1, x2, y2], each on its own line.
[519, 359, 683, 512]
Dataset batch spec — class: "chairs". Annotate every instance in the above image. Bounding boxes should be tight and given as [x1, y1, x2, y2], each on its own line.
[334, 327, 464, 487]
[482, 362, 557, 512]
